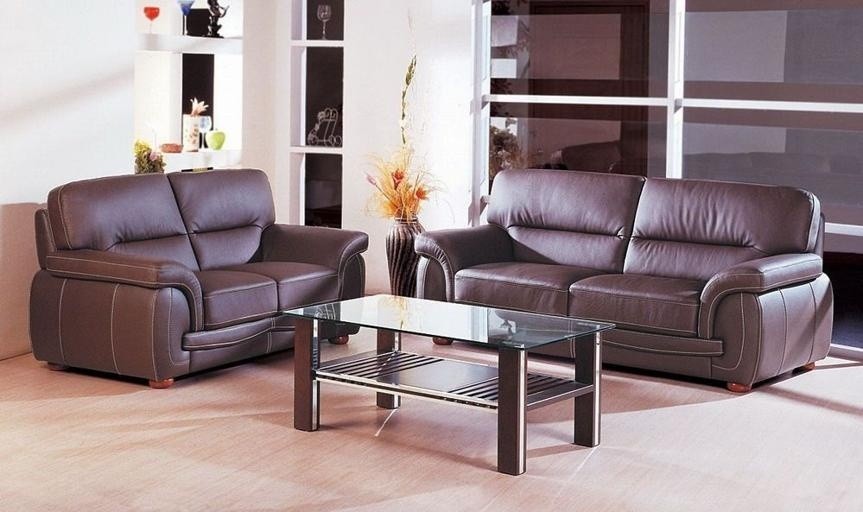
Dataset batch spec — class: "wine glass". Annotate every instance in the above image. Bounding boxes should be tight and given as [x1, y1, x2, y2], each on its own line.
[317, 3, 333, 40]
[142, 6, 161, 34]
[198, 116, 213, 152]
[176, 0, 195, 38]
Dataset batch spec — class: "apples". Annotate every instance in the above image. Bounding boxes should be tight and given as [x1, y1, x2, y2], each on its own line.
[205, 130, 225, 149]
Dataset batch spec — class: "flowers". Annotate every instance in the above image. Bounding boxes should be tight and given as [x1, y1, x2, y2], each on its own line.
[133, 141, 165, 173]
[187, 94, 210, 116]
[363, 50, 448, 225]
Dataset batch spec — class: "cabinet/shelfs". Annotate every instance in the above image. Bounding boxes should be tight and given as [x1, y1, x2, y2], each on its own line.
[290, 38, 342, 155]
[140, 32, 243, 169]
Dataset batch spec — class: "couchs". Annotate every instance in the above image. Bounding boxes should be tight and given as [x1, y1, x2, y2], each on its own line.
[415, 165, 835, 394]
[27, 168, 371, 392]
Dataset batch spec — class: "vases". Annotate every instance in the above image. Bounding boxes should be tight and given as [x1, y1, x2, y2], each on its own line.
[183, 115, 199, 151]
[383, 217, 427, 298]
